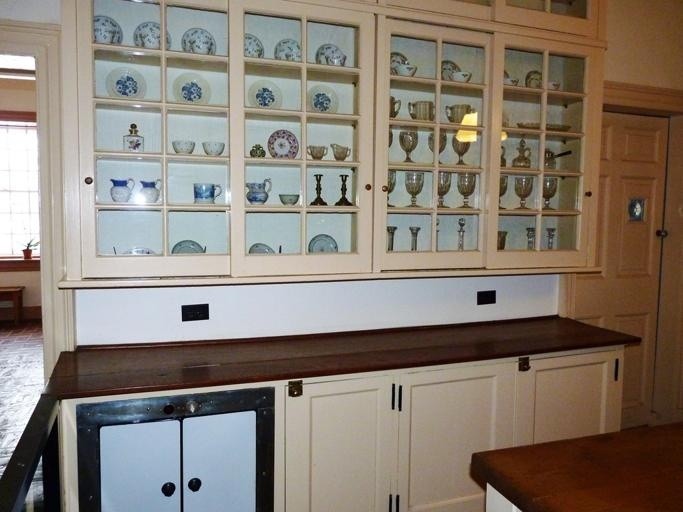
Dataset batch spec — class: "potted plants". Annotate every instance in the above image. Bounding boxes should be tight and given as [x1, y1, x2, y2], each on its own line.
[21, 237, 40, 260]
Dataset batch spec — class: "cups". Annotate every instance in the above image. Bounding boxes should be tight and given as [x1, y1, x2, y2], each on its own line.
[408, 99, 435, 121]
[191, 182, 222, 204]
[389, 95, 401, 118]
[443, 103, 473, 124]
[497, 231, 507, 249]
[329, 143, 350, 161]
[304, 145, 328, 161]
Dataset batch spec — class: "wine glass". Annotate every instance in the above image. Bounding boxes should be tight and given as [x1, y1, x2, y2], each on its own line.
[403, 171, 424, 208]
[455, 172, 475, 209]
[386, 169, 396, 207]
[514, 175, 532, 209]
[542, 176, 558, 210]
[398, 130, 417, 164]
[498, 174, 508, 209]
[425, 132, 446, 165]
[432, 171, 450, 209]
[450, 134, 470, 166]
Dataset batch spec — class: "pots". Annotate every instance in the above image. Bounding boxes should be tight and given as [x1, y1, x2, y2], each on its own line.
[543, 147, 571, 169]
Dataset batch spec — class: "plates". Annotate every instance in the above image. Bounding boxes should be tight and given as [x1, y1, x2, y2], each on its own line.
[314, 44, 346, 65]
[266, 128, 299, 161]
[273, 38, 301, 62]
[91, 15, 122, 48]
[516, 120, 541, 129]
[308, 234, 338, 252]
[121, 247, 157, 255]
[440, 60, 461, 82]
[248, 242, 275, 254]
[180, 27, 217, 55]
[170, 71, 211, 104]
[305, 84, 339, 114]
[103, 66, 146, 101]
[246, 78, 283, 109]
[545, 124, 571, 131]
[504, 71, 509, 80]
[524, 70, 542, 89]
[389, 50, 408, 76]
[133, 20, 172, 51]
[243, 32, 263, 58]
[170, 239, 204, 254]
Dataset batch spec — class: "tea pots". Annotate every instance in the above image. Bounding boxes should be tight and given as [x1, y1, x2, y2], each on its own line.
[244, 178, 272, 207]
[109, 177, 135, 204]
[511, 139, 533, 168]
[138, 178, 162, 203]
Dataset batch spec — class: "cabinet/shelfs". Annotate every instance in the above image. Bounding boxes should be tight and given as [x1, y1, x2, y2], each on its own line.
[66, 3, 237, 285]
[378, 0, 600, 38]
[291, 357, 513, 512]
[517, 354, 629, 447]
[491, 24, 610, 273]
[237, 2, 492, 278]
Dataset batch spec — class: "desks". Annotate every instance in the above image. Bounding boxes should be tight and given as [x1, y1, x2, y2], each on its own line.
[470, 423, 683, 512]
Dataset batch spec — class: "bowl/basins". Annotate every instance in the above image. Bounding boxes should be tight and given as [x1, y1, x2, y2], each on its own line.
[394, 63, 416, 77]
[278, 193, 300, 206]
[547, 81, 560, 90]
[450, 70, 470, 83]
[172, 141, 194, 155]
[505, 77, 519, 86]
[200, 141, 225, 156]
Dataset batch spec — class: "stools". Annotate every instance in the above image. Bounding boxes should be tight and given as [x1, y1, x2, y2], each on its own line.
[0, 287, 23, 326]
